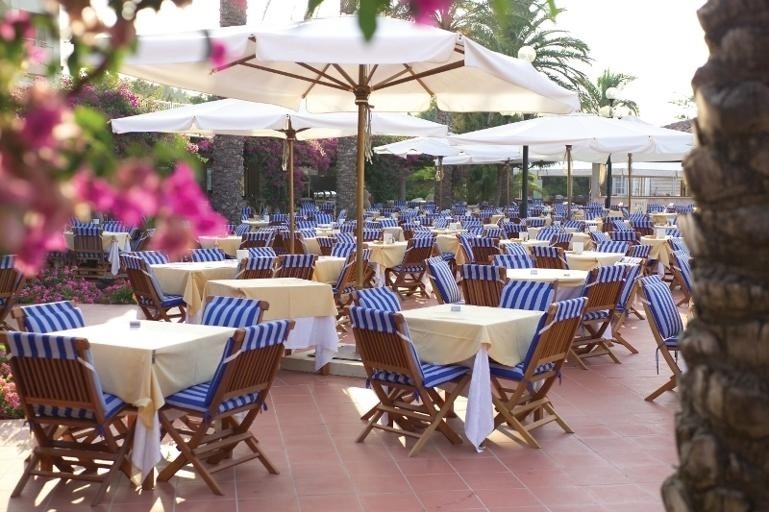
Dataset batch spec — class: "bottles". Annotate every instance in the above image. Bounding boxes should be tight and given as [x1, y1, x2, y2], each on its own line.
[528, 235, 529, 240]
[379, 238, 381, 245]
[524, 237, 525, 241]
[393, 237, 395, 245]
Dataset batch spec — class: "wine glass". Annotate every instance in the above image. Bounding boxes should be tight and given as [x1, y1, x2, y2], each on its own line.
[224, 225, 236, 236]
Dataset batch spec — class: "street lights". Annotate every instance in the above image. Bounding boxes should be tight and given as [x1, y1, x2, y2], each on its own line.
[516, 44, 539, 220]
[603, 85, 618, 207]
